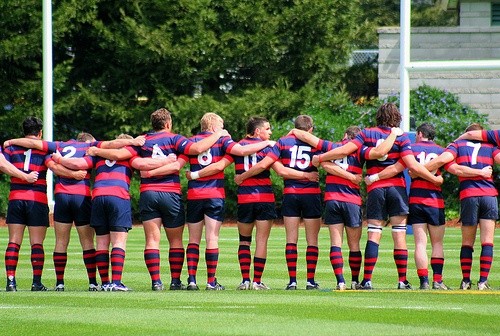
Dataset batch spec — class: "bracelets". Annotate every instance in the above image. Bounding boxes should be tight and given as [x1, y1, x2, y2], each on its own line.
[190, 171, 199, 180]
[369, 174, 380, 183]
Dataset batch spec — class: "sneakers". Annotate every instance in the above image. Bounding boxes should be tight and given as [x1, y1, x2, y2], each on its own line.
[205, 280, 225, 291]
[236, 281, 250, 290]
[397, 281, 416, 290]
[5, 283, 18, 291]
[356, 280, 373, 289]
[30, 282, 51, 291]
[419, 280, 429, 290]
[102, 282, 132, 291]
[335, 282, 346, 290]
[169, 280, 187, 290]
[186, 282, 199, 290]
[151, 281, 166, 291]
[286, 282, 298, 290]
[306, 282, 321, 290]
[350, 281, 360, 289]
[54, 282, 65, 292]
[88, 284, 101, 292]
[476, 281, 493, 290]
[432, 280, 451, 290]
[252, 282, 270, 290]
[460, 280, 476, 290]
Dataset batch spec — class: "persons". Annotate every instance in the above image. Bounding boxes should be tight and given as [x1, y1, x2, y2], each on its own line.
[234, 103, 500, 293]
[2, 116, 87, 292]
[4, 131, 147, 292]
[186, 116, 319, 290]
[53, 134, 176, 292]
[140, 113, 277, 290]
[86, 109, 231, 290]
[0, 146, 39, 183]
